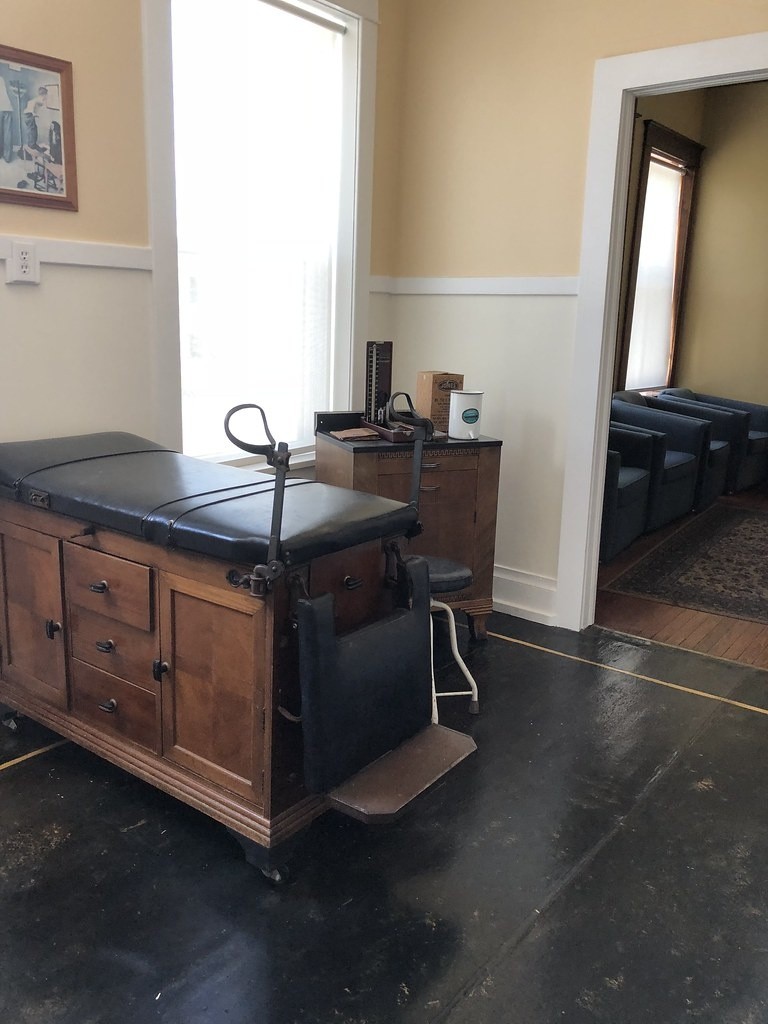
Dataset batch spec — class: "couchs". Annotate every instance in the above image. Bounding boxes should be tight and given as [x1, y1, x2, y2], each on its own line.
[598, 425, 654, 564]
[610, 399, 705, 535]
[658, 387, 768, 496]
[612, 391, 733, 515]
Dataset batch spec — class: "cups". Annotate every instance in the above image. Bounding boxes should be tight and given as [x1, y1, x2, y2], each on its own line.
[448, 389, 485, 440]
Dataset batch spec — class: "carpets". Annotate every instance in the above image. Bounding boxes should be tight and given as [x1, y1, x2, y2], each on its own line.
[599, 503, 768, 625]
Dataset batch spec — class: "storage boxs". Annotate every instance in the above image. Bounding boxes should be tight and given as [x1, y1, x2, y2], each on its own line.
[416, 371, 463, 432]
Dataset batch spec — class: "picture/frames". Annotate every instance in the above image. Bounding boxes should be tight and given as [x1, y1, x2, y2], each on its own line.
[0, 44, 79, 213]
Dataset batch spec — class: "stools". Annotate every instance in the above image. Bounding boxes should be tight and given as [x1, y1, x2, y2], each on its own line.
[406, 555, 485, 723]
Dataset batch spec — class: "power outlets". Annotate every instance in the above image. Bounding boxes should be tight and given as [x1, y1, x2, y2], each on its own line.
[5, 241, 41, 286]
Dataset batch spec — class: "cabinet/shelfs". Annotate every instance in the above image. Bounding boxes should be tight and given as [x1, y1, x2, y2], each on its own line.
[62, 515, 159, 789]
[153, 540, 271, 851]
[0, 498, 71, 746]
[310, 536, 383, 643]
[316, 437, 501, 640]
